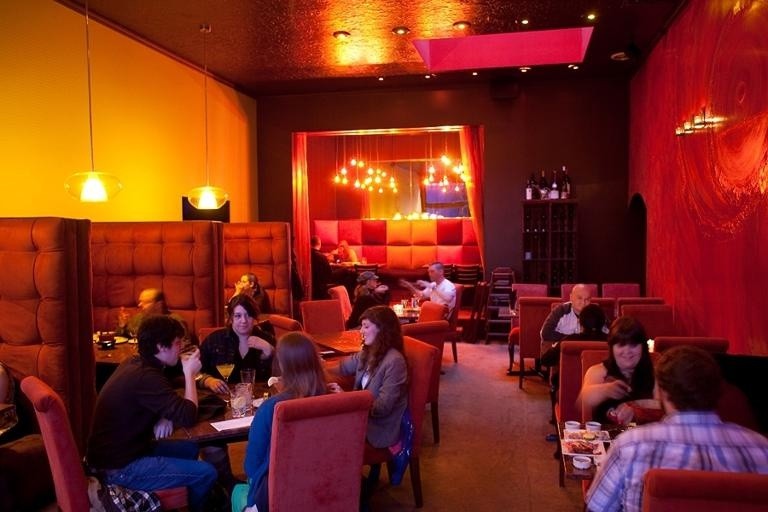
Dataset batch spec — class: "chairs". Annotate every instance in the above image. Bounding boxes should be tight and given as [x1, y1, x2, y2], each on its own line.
[20, 264, 480, 512]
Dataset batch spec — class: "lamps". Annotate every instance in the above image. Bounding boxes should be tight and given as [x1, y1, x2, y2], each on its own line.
[676, 112, 704, 136]
[188, 23, 228, 210]
[65, 1, 122, 203]
[422, 132, 468, 192]
[334, 135, 399, 193]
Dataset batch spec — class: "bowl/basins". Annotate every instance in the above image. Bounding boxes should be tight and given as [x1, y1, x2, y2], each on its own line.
[100, 339, 116, 347]
[573, 456, 592, 469]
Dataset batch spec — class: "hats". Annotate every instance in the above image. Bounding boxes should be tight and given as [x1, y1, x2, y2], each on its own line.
[356, 271, 379, 282]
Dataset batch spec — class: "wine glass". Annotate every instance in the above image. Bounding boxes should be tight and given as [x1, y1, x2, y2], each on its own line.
[216, 360, 235, 385]
[179, 346, 203, 381]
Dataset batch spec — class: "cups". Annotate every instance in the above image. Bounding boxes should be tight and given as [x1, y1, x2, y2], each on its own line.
[565, 421, 581, 429]
[230, 388, 246, 418]
[235, 383, 253, 411]
[241, 368, 255, 397]
[401, 300, 408, 308]
[585, 422, 601, 431]
[411, 298, 419, 307]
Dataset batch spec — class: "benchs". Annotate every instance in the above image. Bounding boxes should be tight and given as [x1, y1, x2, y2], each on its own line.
[0, 217, 293, 482]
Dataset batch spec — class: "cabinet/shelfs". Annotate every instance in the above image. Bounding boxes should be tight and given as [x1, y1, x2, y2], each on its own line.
[485, 267, 516, 345]
[520, 198, 576, 294]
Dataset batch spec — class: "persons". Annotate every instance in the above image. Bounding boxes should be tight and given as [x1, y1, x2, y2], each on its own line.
[291, 234, 456, 329]
[575, 317, 660, 424]
[583, 343, 768, 512]
[539, 284, 610, 402]
[83, 273, 410, 512]
[0, 363, 37, 444]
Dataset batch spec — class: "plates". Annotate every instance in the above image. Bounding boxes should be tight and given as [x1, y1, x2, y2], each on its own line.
[128, 339, 138, 343]
[252, 397, 271, 408]
[563, 429, 611, 443]
[561, 439, 606, 457]
[114, 336, 128, 343]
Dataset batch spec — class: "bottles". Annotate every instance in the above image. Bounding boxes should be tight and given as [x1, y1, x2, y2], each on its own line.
[523, 165, 572, 200]
